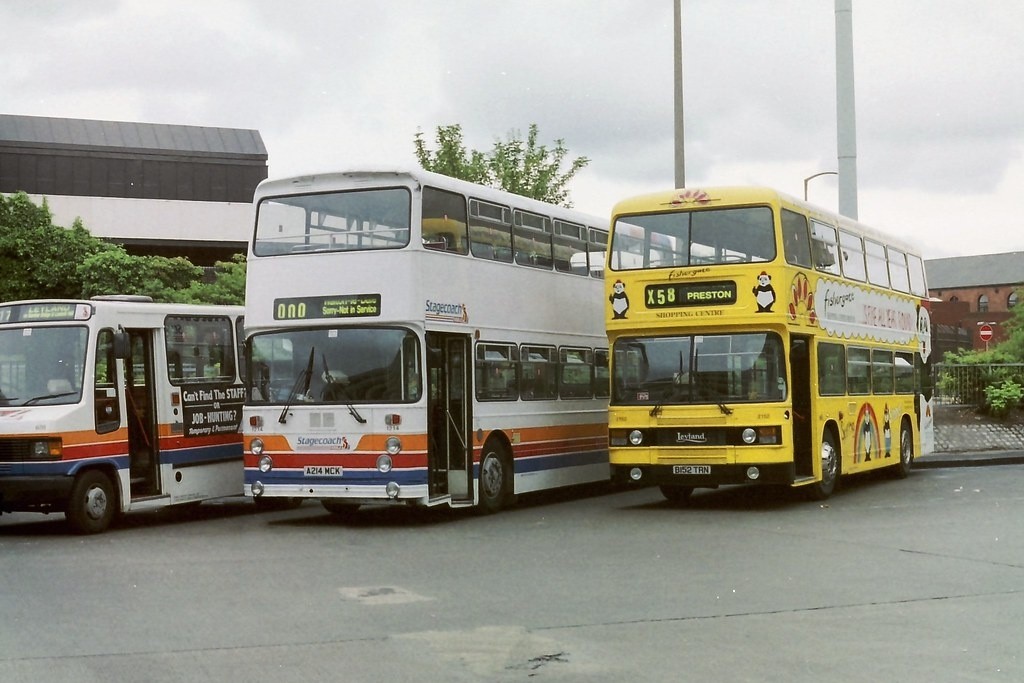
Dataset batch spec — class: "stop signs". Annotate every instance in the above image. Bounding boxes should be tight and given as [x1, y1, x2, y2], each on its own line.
[980, 323, 993, 342]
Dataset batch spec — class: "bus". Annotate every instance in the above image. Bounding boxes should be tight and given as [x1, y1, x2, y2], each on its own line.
[235, 167, 767, 524]
[604, 184, 934, 504]
[0, 294, 292, 536]
